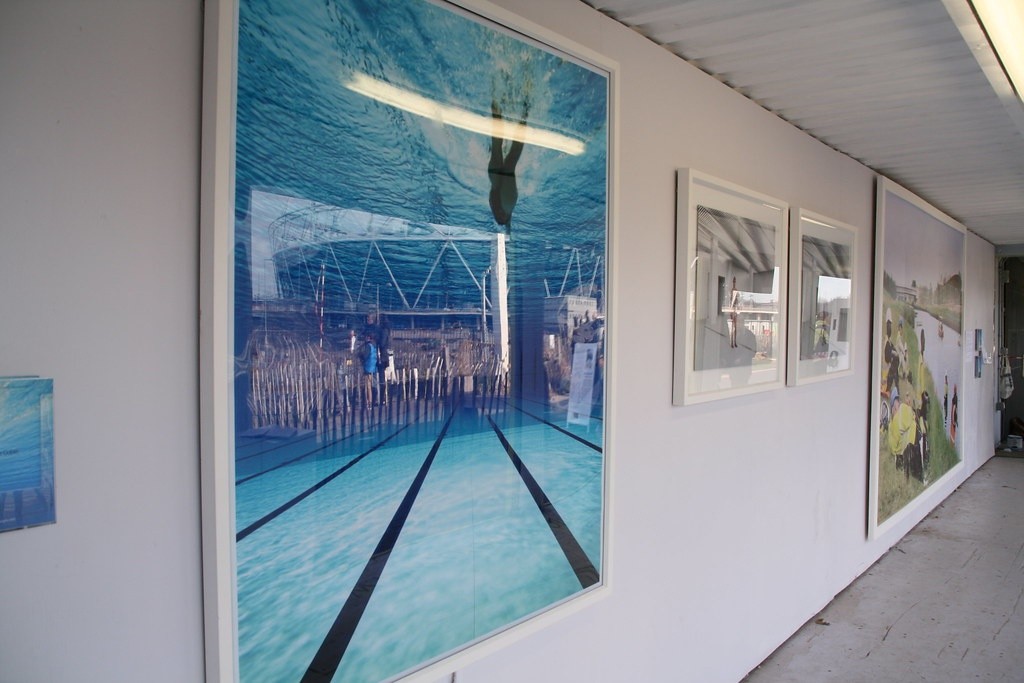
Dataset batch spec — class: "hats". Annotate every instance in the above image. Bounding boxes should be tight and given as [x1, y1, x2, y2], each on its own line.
[890, 387, 900, 406]
[898, 317, 903, 325]
[944, 370, 947, 376]
[886, 308, 892, 322]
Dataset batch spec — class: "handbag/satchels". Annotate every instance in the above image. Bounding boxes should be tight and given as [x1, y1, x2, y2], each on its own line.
[999, 354, 1014, 399]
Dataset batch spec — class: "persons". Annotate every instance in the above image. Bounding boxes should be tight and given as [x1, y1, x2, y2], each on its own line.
[730, 276, 739, 348]
[813, 311, 831, 357]
[880, 307, 933, 486]
[584, 349, 592, 369]
[357, 313, 391, 410]
[349, 330, 356, 354]
[943, 375, 950, 429]
[950, 384, 959, 446]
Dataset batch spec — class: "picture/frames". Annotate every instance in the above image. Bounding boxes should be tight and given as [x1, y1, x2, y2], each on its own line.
[867, 172, 966, 540]
[673, 167, 789, 405]
[787, 207, 856, 390]
[196, 0, 620, 683]
